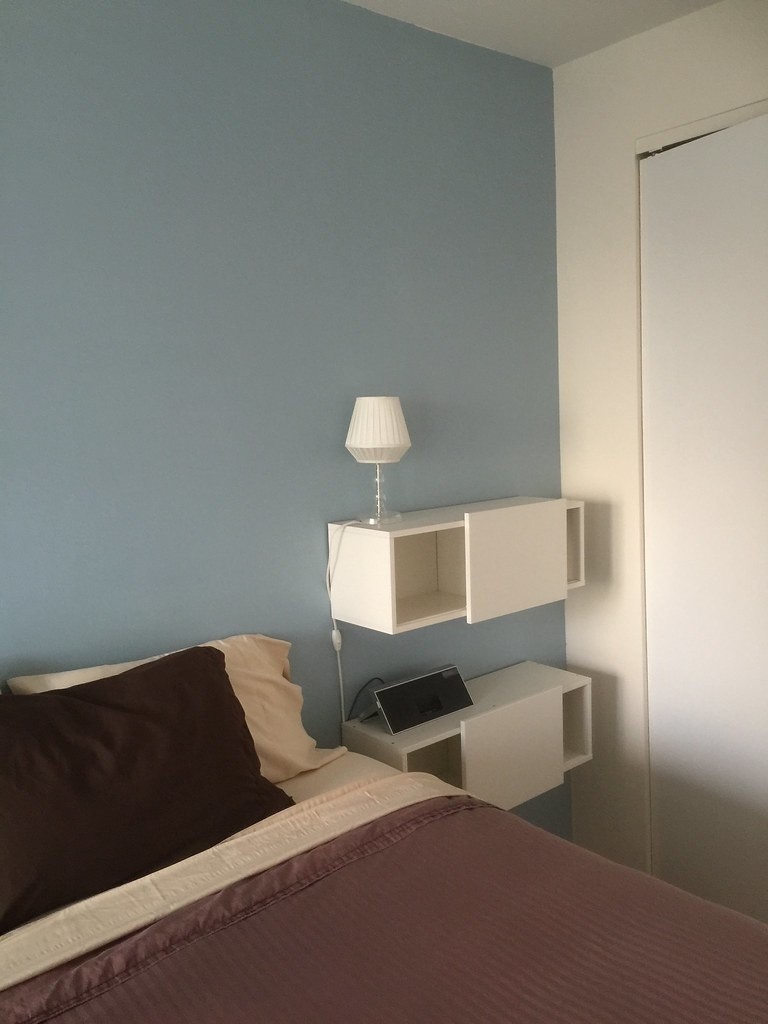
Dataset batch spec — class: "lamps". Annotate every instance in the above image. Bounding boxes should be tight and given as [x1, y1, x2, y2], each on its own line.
[344, 396, 411, 526]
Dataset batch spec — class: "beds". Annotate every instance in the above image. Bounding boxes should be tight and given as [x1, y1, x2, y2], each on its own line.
[1, 749, 768, 1024]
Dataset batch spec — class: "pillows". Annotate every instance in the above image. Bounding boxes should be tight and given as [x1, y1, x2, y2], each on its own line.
[7, 633, 348, 785]
[1, 646, 298, 939]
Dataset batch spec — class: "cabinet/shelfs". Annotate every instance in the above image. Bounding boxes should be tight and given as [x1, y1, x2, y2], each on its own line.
[328, 495, 586, 635]
[342, 658, 594, 811]
